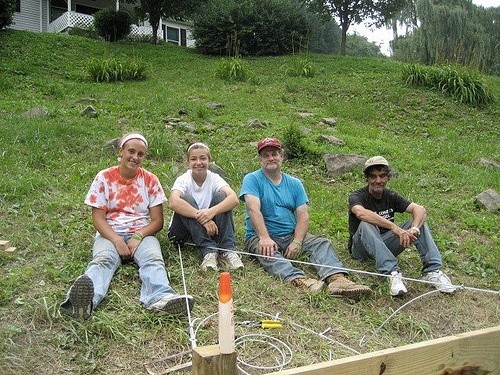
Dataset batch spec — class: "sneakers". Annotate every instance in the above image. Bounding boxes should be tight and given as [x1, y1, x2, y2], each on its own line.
[421, 270, 456, 294]
[326, 274, 372, 299]
[219, 251, 244, 269]
[291, 278, 325, 293]
[200, 252, 218, 271]
[388, 271, 407, 296]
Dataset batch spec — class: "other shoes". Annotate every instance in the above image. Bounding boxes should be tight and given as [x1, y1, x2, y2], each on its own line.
[69, 274, 94, 320]
[145, 295, 195, 318]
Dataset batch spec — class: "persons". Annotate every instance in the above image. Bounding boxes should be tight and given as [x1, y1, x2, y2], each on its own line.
[60, 133, 194, 324]
[239, 137, 371, 300]
[348, 156, 456, 296]
[167, 141, 246, 272]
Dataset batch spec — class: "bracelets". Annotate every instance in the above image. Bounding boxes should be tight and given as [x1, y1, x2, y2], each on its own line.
[132, 234, 142, 241]
[411, 227, 420, 235]
[293, 239, 302, 246]
[135, 231, 144, 238]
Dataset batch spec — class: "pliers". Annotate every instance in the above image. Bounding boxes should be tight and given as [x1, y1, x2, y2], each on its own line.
[236, 319, 283, 328]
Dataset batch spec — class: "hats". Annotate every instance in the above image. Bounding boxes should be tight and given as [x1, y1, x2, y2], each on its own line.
[363, 155, 389, 173]
[257, 138, 282, 153]
